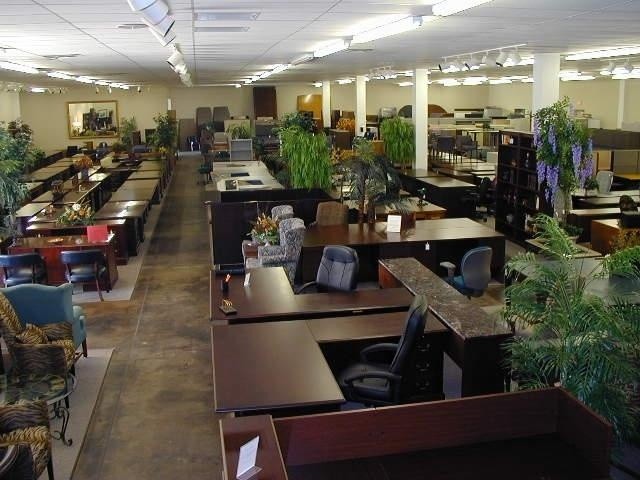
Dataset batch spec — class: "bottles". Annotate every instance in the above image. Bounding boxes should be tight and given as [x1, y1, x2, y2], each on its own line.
[509, 135, 514, 145]
[525, 153, 531, 169]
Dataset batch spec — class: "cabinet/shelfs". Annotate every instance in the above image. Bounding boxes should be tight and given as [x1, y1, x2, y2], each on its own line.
[379, 258, 513, 398]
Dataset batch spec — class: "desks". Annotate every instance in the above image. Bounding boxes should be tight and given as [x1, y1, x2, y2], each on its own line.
[0, 147, 173, 292]
[210, 309, 451, 417]
[0, 374, 75, 447]
[199, 105, 639, 279]
[207, 266, 414, 325]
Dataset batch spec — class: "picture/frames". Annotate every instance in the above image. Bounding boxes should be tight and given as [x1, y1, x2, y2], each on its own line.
[65, 100, 121, 139]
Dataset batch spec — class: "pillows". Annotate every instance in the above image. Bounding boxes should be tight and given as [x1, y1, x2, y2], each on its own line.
[13, 321, 46, 345]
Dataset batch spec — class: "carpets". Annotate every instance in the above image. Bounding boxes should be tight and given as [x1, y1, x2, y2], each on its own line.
[0, 347, 115, 480]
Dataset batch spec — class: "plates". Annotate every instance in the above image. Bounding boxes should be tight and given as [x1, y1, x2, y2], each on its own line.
[49, 238, 64, 243]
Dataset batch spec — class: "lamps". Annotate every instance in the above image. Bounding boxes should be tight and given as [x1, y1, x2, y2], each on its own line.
[125, 0, 191, 73]
[435, 48, 527, 77]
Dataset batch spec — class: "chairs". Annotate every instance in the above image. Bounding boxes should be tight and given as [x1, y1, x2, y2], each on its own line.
[0, 294, 76, 383]
[61, 251, 110, 302]
[294, 245, 359, 297]
[0, 282, 90, 358]
[439, 246, 492, 301]
[0, 254, 49, 288]
[0, 398, 56, 480]
[338, 295, 429, 408]
